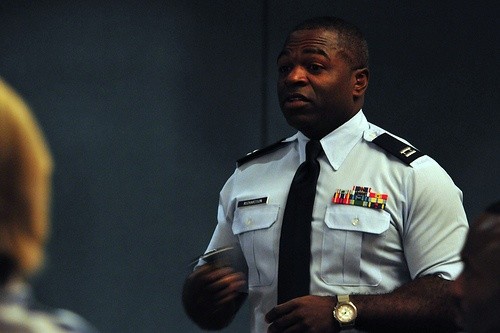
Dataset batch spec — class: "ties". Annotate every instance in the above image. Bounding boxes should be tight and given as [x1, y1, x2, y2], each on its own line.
[276, 139, 325, 306]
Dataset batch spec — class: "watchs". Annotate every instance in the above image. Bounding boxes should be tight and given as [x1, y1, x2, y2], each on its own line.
[332, 294, 358, 333]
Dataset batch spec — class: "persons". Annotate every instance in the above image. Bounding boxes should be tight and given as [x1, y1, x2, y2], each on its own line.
[0, 76, 99, 333]
[447, 200, 500, 333]
[181, 15, 470, 333]
